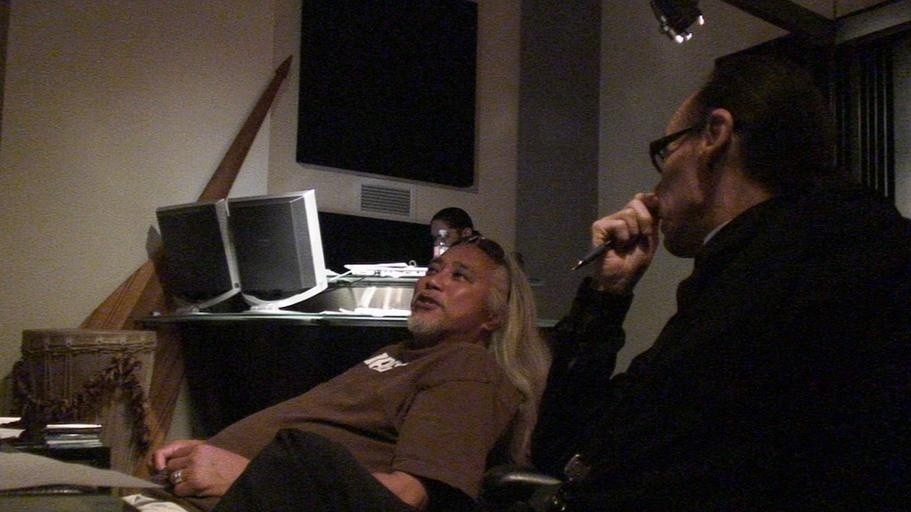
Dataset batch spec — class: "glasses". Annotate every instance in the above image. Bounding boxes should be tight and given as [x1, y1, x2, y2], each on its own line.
[649, 124, 703, 173]
[451, 235, 504, 259]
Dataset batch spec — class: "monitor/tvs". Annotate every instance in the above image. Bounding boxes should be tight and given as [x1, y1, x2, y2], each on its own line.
[154, 199, 241, 314]
[228, 189, 328, 315]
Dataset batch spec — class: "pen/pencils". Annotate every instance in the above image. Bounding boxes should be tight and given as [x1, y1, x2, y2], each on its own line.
[568, 206, 657, 273]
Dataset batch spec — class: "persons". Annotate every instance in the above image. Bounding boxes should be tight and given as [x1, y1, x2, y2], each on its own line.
[530, 49, 910, 512]
[121, 234, 551, 512]
[430, 207, 483, 259]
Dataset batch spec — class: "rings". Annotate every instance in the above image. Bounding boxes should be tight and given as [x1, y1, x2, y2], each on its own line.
[170, 468, 184, 485]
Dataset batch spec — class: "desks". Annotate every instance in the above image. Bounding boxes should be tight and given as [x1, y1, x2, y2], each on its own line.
[132, 311, 406, 437]
[0, 443, 200, 512]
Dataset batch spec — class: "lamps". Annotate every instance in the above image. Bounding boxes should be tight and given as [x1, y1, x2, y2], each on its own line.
[650, 1, 705, 44]
